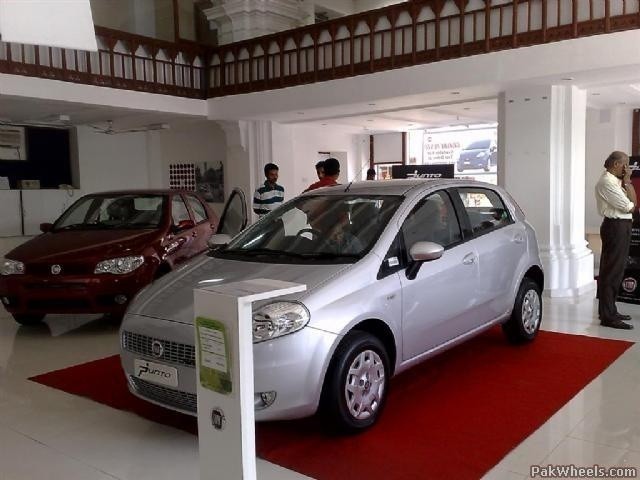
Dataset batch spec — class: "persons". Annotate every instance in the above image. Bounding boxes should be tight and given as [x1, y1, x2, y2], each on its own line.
[321, 216, 364, 256]
[351, 169, 377, 224]
[252, 163, 285, 219]
[294, 158, 350, 239]
[315, 160, 326, 180]
[594, 150, 638, 330]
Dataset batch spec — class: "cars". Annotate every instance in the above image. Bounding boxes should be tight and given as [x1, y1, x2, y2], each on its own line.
[457, 139, 497, 172]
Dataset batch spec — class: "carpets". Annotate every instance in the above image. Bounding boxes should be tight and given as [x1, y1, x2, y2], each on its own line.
[26, 323, 638, 479]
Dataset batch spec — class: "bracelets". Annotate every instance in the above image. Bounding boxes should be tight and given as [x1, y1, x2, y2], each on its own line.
[624, 182, 633, 186]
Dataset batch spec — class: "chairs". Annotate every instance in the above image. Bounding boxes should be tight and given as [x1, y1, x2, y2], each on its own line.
[103, 199, 135, 225]
[405, 199, 453, 252]
[345, 201, 378, 247]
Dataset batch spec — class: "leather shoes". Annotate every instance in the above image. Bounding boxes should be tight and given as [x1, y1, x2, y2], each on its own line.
[600, 317, 634, 330]
[598, 312, 632, 320]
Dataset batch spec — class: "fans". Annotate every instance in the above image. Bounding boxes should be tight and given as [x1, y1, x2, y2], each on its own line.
[88, 120, 147, 135]
